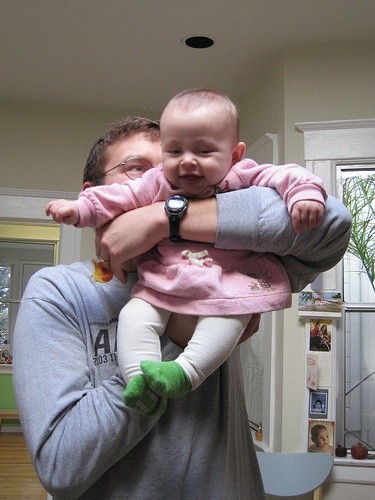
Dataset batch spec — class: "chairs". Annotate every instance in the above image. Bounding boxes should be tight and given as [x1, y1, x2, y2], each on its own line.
[256, 451, 334, 500]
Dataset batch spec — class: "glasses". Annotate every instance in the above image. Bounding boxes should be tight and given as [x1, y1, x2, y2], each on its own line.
[104, 157, 154, 180]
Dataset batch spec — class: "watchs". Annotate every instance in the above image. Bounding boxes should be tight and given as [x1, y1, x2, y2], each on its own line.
[164, 193, 188, 242]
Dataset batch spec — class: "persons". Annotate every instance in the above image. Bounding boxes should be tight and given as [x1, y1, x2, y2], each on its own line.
[46, 88, 328, 419]
[301, 292, 331, 351]
[308, 397, 333, 454]
[12, 114, 352, 500]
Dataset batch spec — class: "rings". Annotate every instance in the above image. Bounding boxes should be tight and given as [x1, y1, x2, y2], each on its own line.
[100, 256, 110, 262]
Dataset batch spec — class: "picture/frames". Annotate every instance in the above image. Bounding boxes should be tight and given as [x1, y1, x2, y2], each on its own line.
[308, 318, 333, 354]
[309, 389, 328, 419]
[298, 290, 343, 312]
[308, 420, 334, 456]
